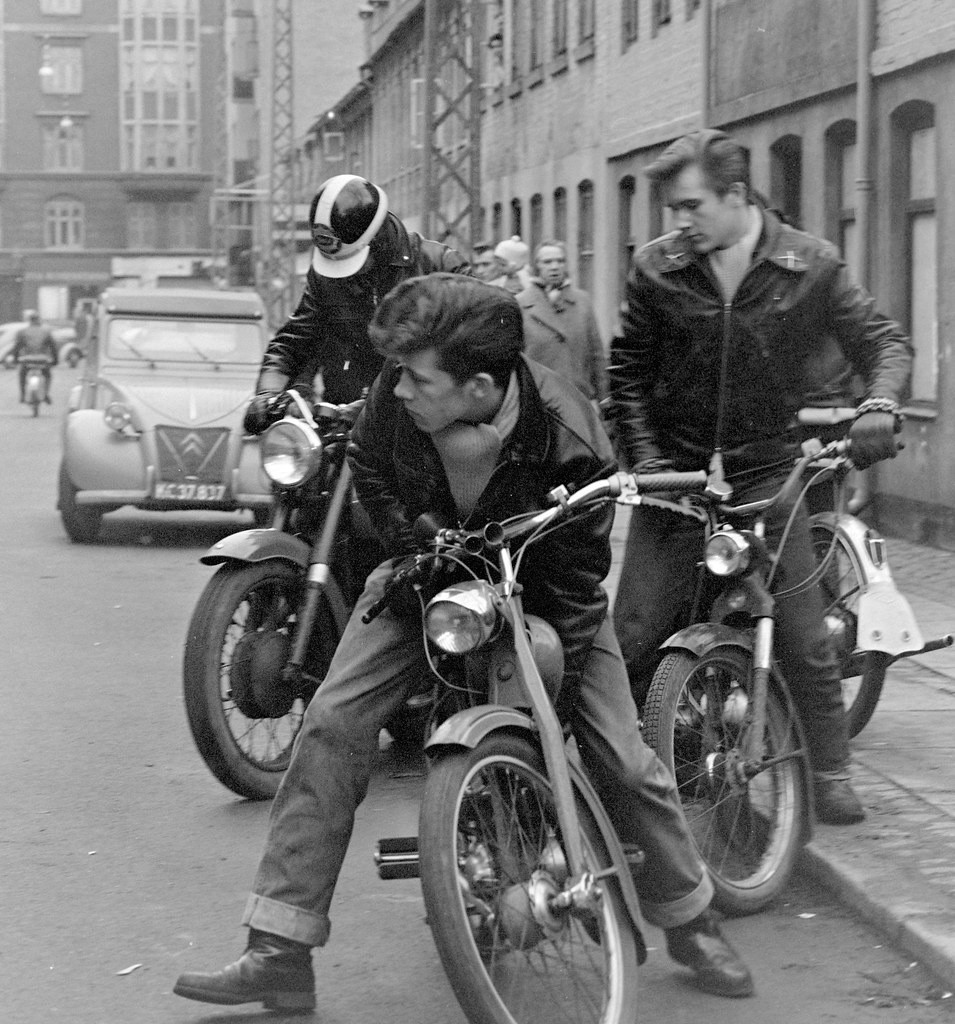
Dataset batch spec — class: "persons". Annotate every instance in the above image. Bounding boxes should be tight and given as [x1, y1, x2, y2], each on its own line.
[531, 241, 609, 411]
[605, 127, 916, 823]
[172, 273, 753, 1008]
[243, 175, 475, 435]
[472, 236, 570, 381]
[13, 314, 59, 405]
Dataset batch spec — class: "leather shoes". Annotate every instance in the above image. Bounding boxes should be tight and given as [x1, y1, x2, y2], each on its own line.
[665, 910, 754, 995]
[173, 932, 317, 1009]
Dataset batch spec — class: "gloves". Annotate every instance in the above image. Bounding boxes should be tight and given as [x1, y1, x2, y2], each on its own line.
[850, 413, 898, 469]
[636, 460, 683, 502]
[385, 558, 425, 615]
[244, 392, 282, 434]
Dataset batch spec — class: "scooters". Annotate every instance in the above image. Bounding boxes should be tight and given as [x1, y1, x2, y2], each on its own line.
[7, 353, 59, 418]
[359, 450, 712, 1024]
[636, 421, 955, 916]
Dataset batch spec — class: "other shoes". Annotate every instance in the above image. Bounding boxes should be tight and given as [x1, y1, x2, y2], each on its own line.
[815, 780, 864, 819]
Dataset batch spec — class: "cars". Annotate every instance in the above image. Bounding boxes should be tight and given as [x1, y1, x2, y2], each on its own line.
[52, 284, 280, 544]
[0, 316, 84, 371]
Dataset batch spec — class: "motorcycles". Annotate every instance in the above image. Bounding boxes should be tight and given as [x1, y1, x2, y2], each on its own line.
[179, 383, 439, 802]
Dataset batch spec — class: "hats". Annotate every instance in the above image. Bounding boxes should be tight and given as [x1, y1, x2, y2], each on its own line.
[495, 236, 529, 265]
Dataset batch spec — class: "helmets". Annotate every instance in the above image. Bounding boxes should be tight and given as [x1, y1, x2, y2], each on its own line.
[311, 175, 387, 278]
